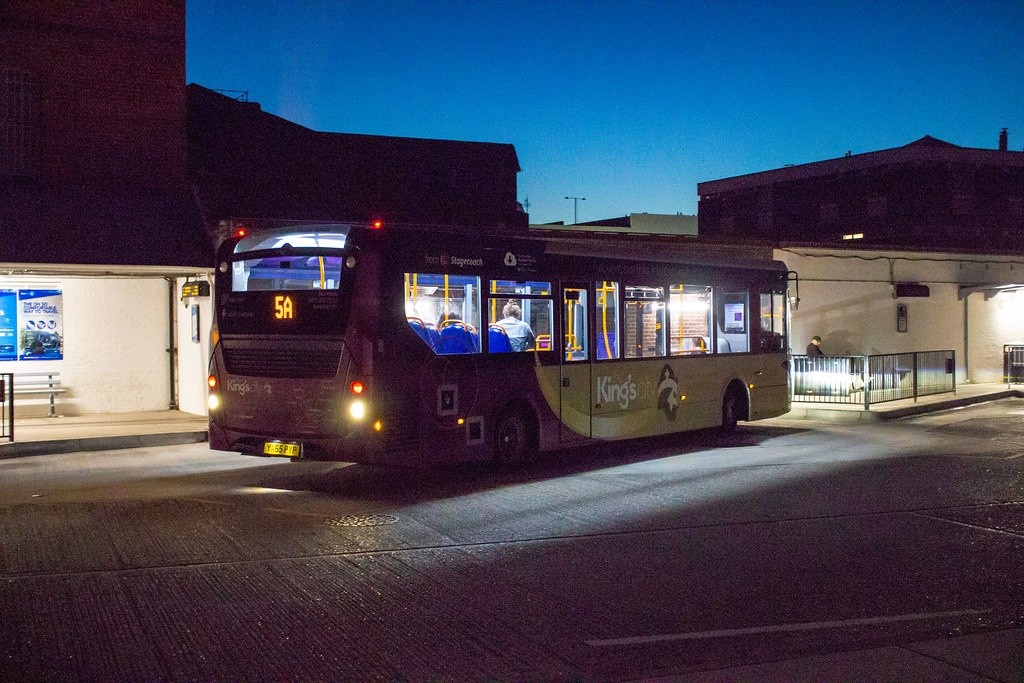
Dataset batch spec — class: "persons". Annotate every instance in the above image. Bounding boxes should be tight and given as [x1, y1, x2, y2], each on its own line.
[807, 336, 825, 358]
[492, 301, 536, 352]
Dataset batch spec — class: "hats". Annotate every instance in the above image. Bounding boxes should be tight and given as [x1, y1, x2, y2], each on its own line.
[811, 336, 821, 342]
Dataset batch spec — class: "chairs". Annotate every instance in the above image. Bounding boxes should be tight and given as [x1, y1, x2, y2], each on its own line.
[597, 331, 616, 358]
[407, 317, 514, 353]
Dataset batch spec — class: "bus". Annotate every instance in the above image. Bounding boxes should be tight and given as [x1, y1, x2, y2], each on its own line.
[207, 220, 800, 471]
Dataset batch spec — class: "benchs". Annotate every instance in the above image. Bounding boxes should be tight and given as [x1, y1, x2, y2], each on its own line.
[0, 371, 66, 419]
